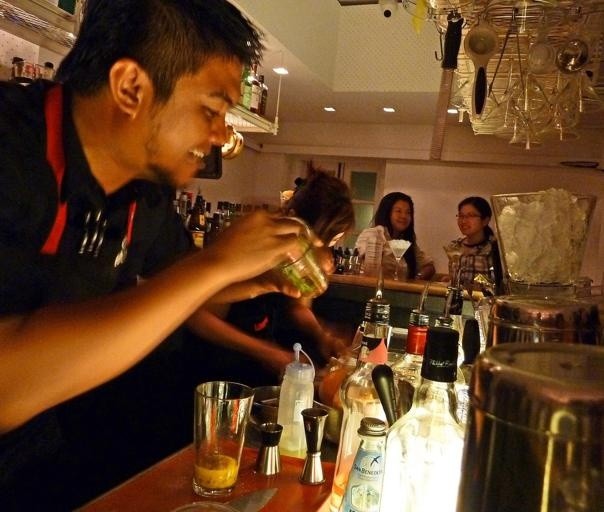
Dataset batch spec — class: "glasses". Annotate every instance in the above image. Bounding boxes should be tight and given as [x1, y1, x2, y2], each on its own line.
[455, 212, 481, 220]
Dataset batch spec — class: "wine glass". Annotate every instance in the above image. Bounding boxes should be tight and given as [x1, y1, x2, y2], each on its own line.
[385, 238, 413, 282]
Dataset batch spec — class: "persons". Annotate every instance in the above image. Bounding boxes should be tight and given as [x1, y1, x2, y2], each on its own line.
[214, 155, 356, 388]
[454, 195, 504, 293]
[1, 0, 326, 511]
[367, 190, 436, 282]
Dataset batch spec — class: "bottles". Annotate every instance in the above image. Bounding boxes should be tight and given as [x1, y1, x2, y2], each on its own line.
[256, 421, 282, 476]
[8, 56, 55, 82]
[317, 292, 603, 512]
[331, 243, 366, 275]
[169, 188, 266, 250]
[240, 62, 269, 119]
[299, 406, 329, 486]
[274, 341, 317, 460]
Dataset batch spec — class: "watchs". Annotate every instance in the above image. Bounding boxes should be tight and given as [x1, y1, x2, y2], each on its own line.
[415, 272, 425, 280]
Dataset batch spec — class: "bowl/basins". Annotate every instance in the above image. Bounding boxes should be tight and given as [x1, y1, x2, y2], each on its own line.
[248, 384, 281, 431]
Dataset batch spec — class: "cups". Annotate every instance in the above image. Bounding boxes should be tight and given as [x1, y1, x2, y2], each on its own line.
[487, 191, 598, 295]
[263, 216, 330, 301]
[190, 380, 256, 497]
